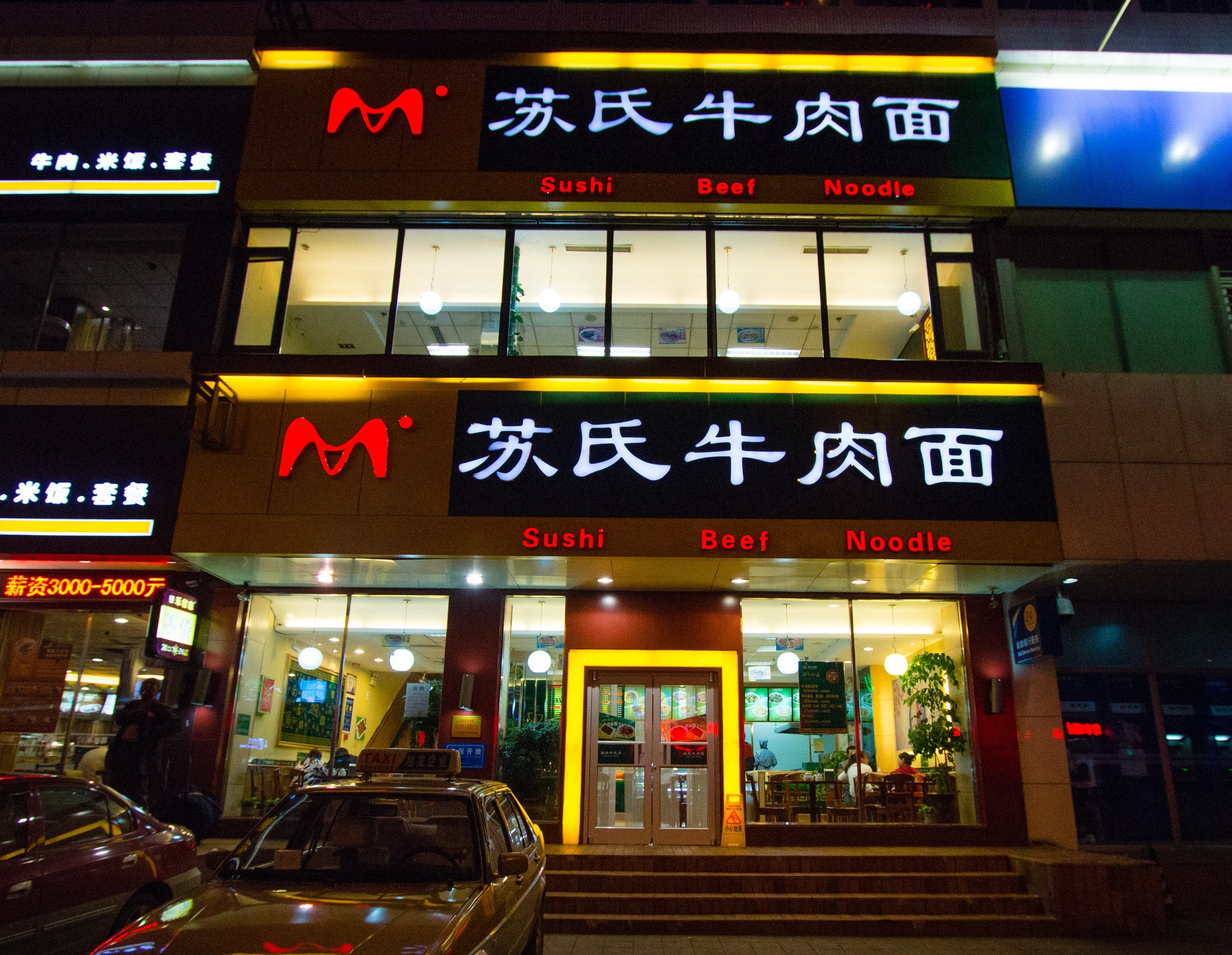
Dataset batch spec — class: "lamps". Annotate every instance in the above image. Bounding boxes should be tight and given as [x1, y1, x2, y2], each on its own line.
[775, 603, 800, 675]
[297, 597, 324, 671]
[896, 248, 922, 317]
[527, 600, 552, 674]
[536, 245, 562, 314]
[882, 604, 909, 676]
[716, 247, 742, 315]
[388, 599, 415, 672]
[417, 243, 444, 317]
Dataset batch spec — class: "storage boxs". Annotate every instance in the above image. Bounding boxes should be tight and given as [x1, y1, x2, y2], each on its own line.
[823, 768, 835, 782]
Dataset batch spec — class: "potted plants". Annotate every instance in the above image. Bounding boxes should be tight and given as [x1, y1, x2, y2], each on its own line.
[496, 719, 562, 822]
[240, 796, 261, 817]
[916, 805, 936, 824]
[899, 651, 968, 825]
[263, 799, 278, 814]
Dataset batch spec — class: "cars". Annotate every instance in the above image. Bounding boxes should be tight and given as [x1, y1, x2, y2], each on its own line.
[94, 745, 546, 955]
[0, 769, 201, 952]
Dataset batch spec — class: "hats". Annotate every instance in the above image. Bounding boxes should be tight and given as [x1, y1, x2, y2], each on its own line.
[759, 739, 768, 745]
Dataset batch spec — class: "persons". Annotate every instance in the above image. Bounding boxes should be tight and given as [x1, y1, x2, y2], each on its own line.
[838, 746, 918, 807]
[290, 747, 356, 789]
[744, 734, 778, 770]
[74, 678, 180, 818]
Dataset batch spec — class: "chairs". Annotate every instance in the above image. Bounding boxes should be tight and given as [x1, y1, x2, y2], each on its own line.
[243, 765, 306, 818]
[305, 812, 510, 878]
[744, 771, 959, 824]
[0, 794, 132, 854]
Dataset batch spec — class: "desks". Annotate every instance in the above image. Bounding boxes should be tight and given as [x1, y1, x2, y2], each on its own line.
[781, 778, 850, 822]
